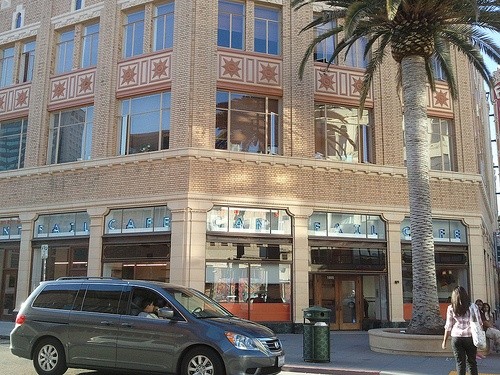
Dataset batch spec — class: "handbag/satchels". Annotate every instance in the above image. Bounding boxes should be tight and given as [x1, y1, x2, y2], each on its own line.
[471, 322, 487, 351]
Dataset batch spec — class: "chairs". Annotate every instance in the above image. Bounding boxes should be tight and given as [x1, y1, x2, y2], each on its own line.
[131, 297, 145, 316]
[156, 295, 164, 307]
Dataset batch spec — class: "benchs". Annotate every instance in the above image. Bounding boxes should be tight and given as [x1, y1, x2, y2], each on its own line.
[368, 327, 490, 356]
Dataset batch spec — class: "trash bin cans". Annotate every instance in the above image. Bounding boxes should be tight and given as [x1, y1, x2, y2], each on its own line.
[302, 306, 331, 363]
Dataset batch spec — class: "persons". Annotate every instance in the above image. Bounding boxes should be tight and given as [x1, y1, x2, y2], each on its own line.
[442, 286, 486, 375]
[138, 299, 159, 320]
[475, 299, 500, 353]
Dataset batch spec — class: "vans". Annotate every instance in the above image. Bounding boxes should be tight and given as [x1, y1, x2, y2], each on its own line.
[9, 280, 284, 375]
[57, 276, 125, 280]
